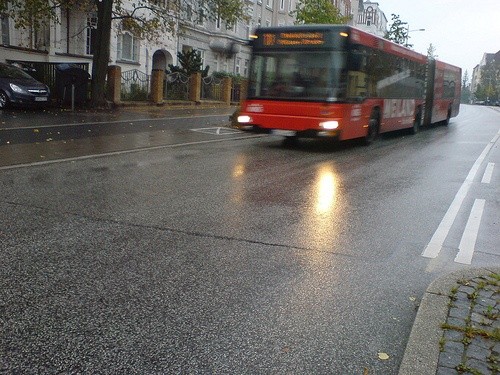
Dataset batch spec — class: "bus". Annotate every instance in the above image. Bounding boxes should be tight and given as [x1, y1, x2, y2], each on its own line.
[234, 24, 461, 144]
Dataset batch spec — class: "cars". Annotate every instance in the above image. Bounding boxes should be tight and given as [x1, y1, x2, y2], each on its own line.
[0, 63, 51, 111]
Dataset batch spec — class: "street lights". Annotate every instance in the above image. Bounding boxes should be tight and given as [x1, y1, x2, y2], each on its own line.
[405, 24, 425, 47]
